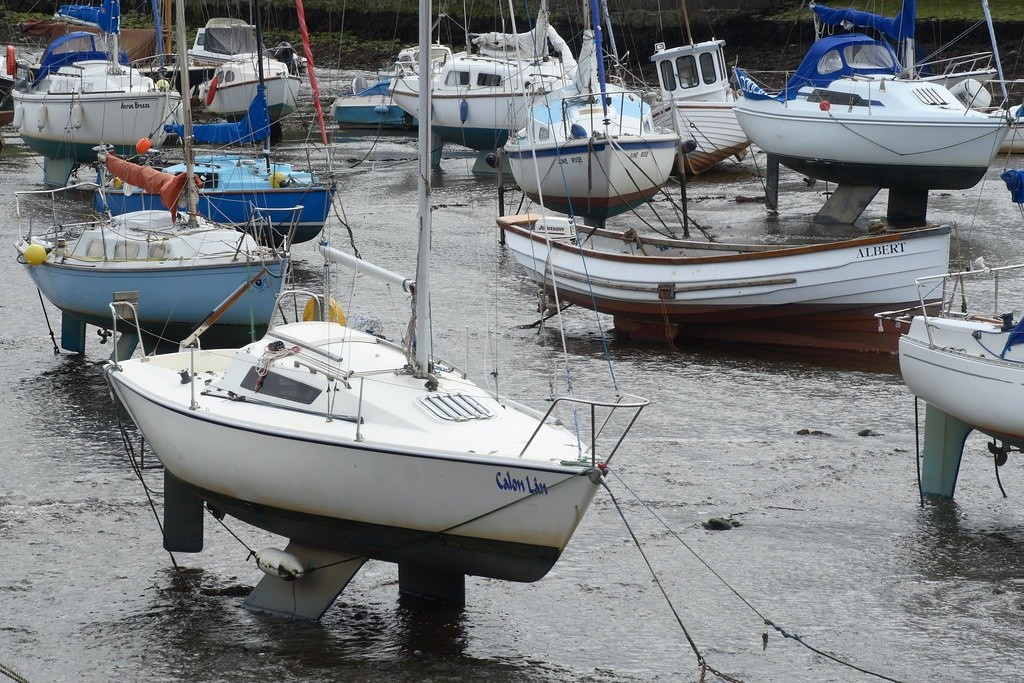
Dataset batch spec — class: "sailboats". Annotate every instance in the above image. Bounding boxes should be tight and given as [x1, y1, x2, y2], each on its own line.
[0, 0, 1024, 621]
